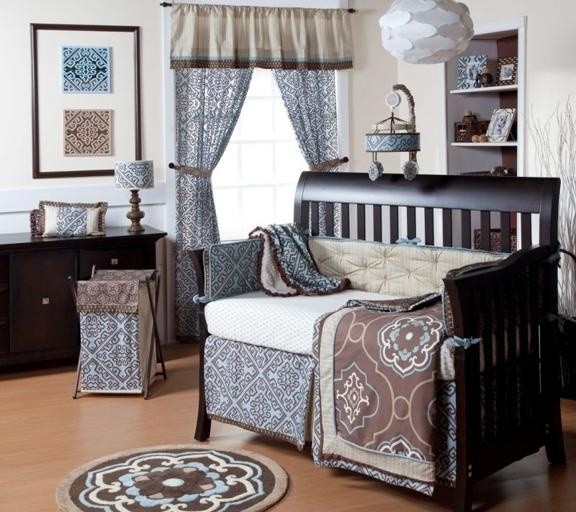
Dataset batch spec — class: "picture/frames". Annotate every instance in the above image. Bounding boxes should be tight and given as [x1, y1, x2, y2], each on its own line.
[486, 109, 516, 142]
[30, 23, 141, 179]
[496, 57, 517, 85]
[457, 54, 487, 89]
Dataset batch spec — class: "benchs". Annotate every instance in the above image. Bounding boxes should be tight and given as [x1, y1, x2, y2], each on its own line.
[195, 171, 566, 512]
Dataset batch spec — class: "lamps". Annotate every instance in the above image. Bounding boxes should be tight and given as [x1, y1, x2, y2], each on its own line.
[380, 1, 475, 64]
[115, 159, 155, 232]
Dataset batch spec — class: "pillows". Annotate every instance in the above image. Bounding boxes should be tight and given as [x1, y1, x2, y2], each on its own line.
[38, 201, 106, 236]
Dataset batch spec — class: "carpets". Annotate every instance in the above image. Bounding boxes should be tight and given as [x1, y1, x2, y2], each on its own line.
[56, 444, 288, 512]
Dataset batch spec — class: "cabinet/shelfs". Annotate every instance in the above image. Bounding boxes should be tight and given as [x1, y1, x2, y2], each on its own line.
[0, 225, 167, 375]
[437, 17, 527, 179]
